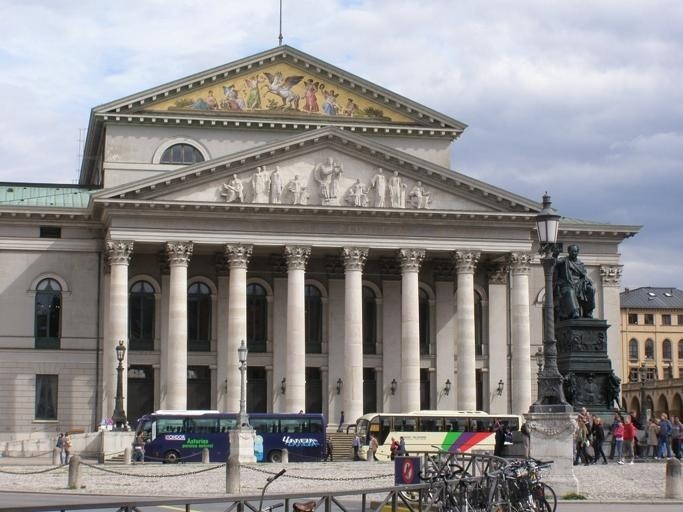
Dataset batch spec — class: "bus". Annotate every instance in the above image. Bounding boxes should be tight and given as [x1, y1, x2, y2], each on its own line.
[347, 410, 527, 462]
[133, 409, 326, 465]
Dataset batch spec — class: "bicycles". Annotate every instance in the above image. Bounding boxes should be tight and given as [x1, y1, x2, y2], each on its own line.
[420, 445, 557, 512]
[259, 467, 315, 511]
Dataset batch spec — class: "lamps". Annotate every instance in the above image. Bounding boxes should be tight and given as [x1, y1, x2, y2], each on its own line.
[217, 377, 508, 397]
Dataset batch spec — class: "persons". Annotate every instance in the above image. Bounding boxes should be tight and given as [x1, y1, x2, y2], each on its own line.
[352, 433, 359, 460]
[390, 419, 514, 461]
[323, 436, 333, 462]
[132, 431, 145, 464]
[573, 407, 683, 466]
[555, 245, 596, 320]
[220, 157, 431, 209]
[65, 431, 72, 465]
[369, 433, 378, 461]
[337, 411, 344, 432]
[174, 73, 358, 115]
[56, 433, 64, 464]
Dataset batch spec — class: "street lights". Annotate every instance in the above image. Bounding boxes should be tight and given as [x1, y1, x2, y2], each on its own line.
[527, 190, 575, 413]
[111, 339, 128, 429]
[236, 338, 251, 430]
[638, 361, 649, 425]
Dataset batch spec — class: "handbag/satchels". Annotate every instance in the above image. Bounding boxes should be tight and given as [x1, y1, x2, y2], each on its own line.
[586, 440, 591, 448]
